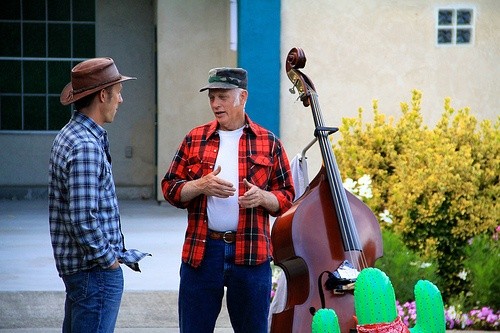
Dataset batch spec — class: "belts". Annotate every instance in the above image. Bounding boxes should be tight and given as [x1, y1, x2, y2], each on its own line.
[207, 230, 236, 244]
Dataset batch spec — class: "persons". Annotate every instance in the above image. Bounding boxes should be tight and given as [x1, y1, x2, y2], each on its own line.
[162, 67, 296, 333]
[47, 57, 153, 333]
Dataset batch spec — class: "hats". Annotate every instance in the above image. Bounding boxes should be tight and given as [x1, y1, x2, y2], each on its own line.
[200, 67, 248, 93]
[61, 57, 138, 105]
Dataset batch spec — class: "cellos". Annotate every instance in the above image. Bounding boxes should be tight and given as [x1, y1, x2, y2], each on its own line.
[270, 46, 384, 333]
